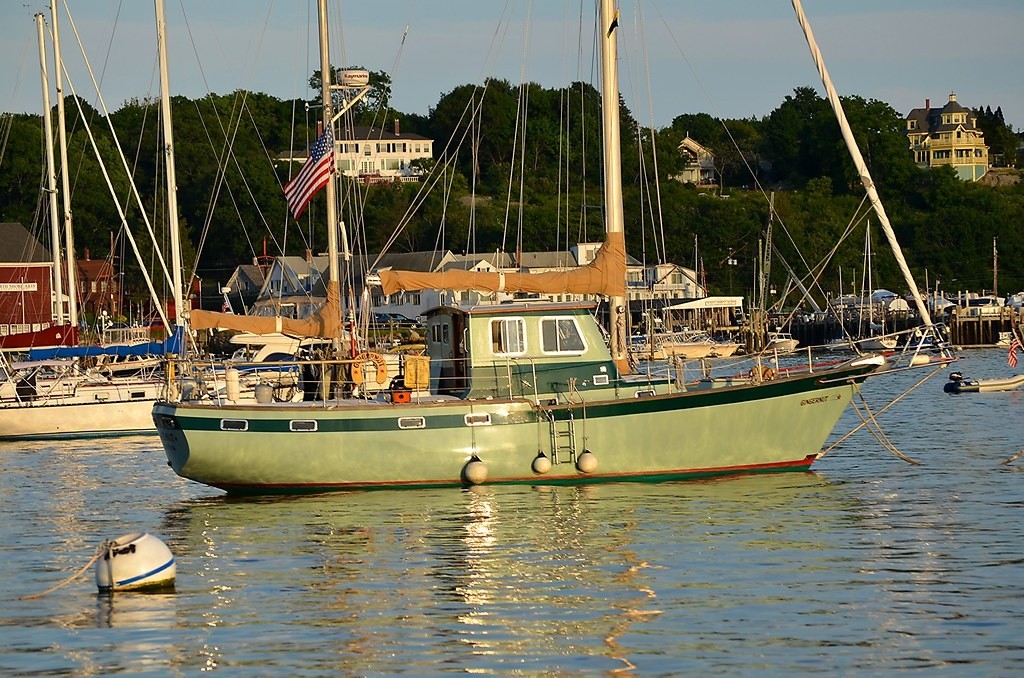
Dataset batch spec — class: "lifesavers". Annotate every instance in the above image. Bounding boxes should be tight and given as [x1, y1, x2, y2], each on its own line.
[164, 353, 174, 381]
[352, 351, 387, 386]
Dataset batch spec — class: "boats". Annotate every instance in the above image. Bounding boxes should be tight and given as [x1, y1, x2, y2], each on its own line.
[943, 370, 1024, 395]
[826, 219, 898, 350]
[644, 342, 745, 359]
[765, 332, 800, 353]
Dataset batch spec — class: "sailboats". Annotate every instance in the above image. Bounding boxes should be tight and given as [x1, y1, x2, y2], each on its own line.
[0, 1, 430, 443]
[151, 0, 961, 494]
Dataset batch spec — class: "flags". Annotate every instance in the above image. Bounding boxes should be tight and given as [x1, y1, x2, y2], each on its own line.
[284, 120, 336, 221]
[1007, 332, 1021, 367]
